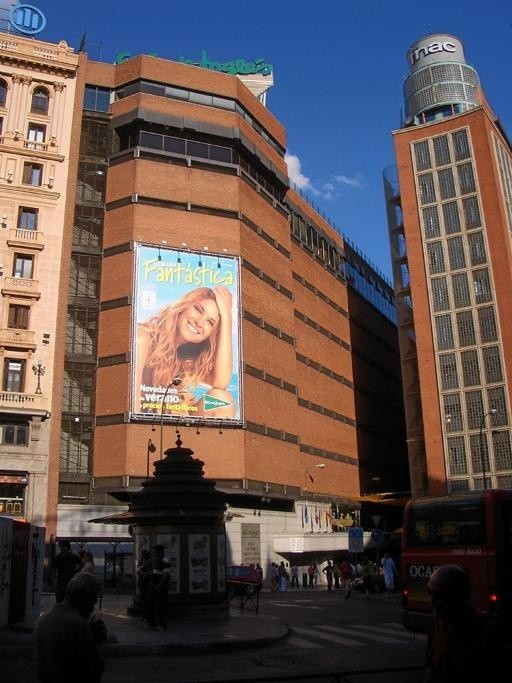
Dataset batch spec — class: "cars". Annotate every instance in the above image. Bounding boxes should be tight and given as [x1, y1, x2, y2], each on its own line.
[225, 563, 263, 596]
[353, 566, 383, 594]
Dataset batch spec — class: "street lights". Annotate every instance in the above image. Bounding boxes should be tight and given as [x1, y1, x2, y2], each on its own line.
[160, 378, 181, 459]
[304, 464, 326, 533]
[479, 409, 497, 490]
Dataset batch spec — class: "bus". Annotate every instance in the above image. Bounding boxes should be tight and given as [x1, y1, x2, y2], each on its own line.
[400, 489, 511, 644]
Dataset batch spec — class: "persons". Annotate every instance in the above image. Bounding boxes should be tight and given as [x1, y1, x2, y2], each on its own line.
[48, 538, 85, 603]
[251, 554, 379, 594]
[33, 571, 105, 683]
[424, 561, 503, 682]
[134, 282, 238, 421]
[134, 543, 171, 632]
[380, 552, 398, 598]
[337, 557, 353, 599]
[80, 551, 95, 573]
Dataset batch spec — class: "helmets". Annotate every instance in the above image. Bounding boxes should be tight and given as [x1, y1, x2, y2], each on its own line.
[422, 565, 473, 608]
